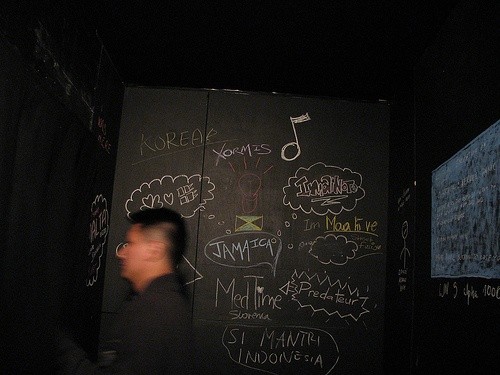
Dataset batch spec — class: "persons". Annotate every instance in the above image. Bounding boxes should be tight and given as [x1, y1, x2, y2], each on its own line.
[53, 208, 198, 374]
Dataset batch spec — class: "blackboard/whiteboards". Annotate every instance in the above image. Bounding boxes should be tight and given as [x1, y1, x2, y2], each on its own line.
[92, 86, 416, 375]
